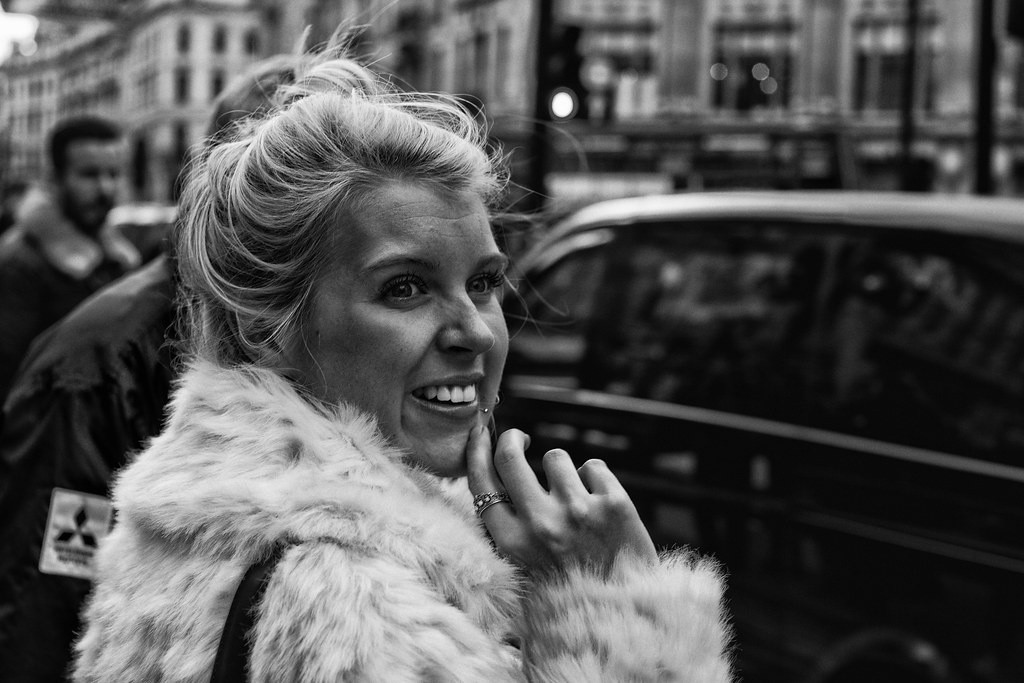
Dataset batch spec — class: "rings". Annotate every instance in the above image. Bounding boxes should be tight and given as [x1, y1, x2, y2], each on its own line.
[472, 490, 510, 517]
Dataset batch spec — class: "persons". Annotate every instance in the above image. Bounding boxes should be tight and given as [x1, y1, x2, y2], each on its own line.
[0, 52, 325, 683]
[70, 8, 738, 683]
[0, 109, 147, 406]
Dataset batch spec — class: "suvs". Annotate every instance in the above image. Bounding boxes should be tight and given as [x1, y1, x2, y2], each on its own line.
[496, 185, 1024, 683]
